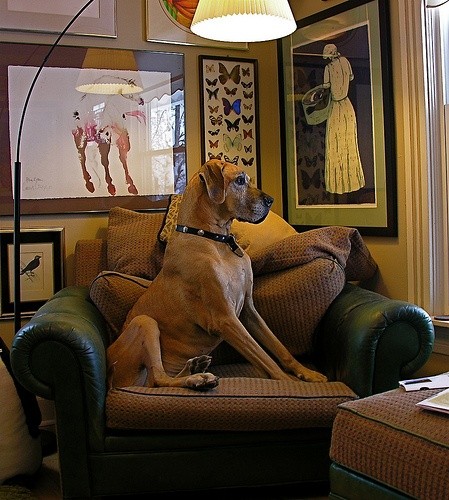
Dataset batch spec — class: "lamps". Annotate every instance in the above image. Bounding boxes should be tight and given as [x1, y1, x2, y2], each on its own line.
[14, 0, 297, 335]
[75, 48, 144, 95]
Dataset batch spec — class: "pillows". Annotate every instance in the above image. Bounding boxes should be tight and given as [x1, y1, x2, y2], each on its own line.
[0, 338, 58, 485]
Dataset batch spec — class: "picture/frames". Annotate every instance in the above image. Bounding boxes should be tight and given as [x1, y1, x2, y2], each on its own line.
[199, 55, 262, 191]
[276, 0, 399, 238]
[144, 0, 249, 51]
[0, 227, 66, 320]
[0, 0, 118, 38]
[0, 41, 187, 217]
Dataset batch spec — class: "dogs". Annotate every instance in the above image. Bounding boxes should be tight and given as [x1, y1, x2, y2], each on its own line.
[107, 160, 330, 389]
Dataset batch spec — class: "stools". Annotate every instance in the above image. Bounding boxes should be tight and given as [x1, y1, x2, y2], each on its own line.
[328, 387, 449, 500]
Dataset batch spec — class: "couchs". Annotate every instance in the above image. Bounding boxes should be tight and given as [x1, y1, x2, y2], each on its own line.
[11, 207, 435, 500]
